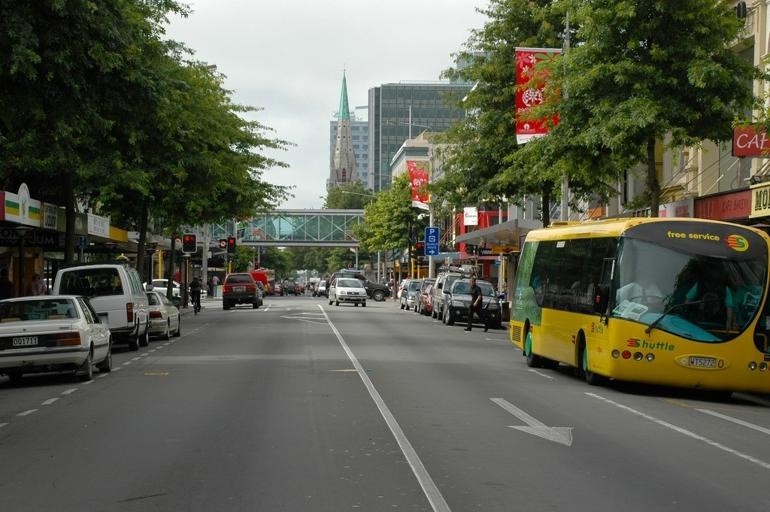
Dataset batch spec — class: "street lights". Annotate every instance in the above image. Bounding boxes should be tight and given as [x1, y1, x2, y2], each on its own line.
[417, 201, 436, 280]
[11, 223, 36, 316]
[341, 190, 378, 199]
[103, 241, 120, 286]
[182, 254, 192, 308]
[146, 247, 157, 302]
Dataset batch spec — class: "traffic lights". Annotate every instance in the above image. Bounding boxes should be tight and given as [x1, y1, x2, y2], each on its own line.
[417, 240, 426, 257]
[220, 239, 226, 248]
[182, 234, 198, 254]
[228, 236, 236, 253]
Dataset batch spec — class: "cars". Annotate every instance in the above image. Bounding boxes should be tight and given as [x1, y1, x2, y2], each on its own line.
[42, 264, 208, 351]
[533, 279, 594, 312]
[0, 295, 112, 384]
[220, 271, 392, 310]
[397, 271, 502, 329]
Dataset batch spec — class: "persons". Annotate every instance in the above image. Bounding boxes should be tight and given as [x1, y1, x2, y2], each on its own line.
[464, 277, 490, 332]
[284, 278, 288, 297]
[0, 268, 16, 319]
[682, 267, 734, 330]
[734, 269, 764, 331]
[188, 276, 202, 312]
[26, 274, 49, 306]
[380, 276, 401, 299]
[615, 266, 670, 305]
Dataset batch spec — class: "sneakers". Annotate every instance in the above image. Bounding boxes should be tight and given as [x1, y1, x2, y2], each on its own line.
[484, 324, 489, 332]
[464, 327, 472, 332]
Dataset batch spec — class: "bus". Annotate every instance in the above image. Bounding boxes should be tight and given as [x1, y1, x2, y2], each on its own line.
[508, 216, 770, 398]
